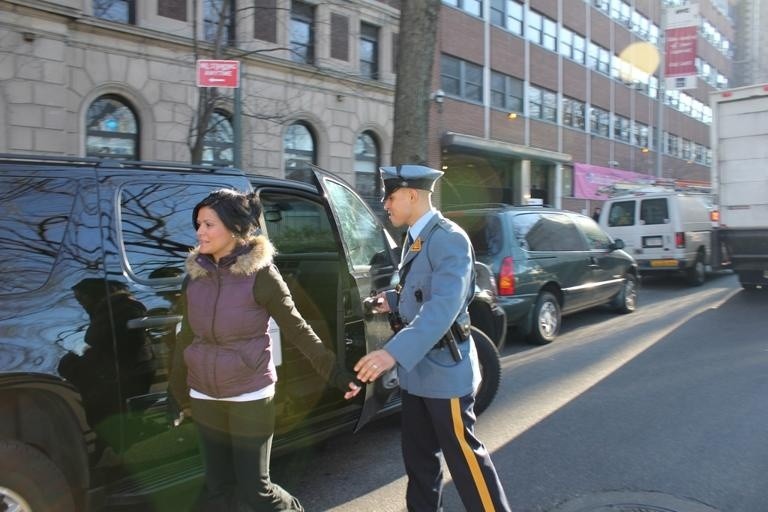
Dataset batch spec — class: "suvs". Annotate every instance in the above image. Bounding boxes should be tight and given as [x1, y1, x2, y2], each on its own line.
[1, 152, 508, 512]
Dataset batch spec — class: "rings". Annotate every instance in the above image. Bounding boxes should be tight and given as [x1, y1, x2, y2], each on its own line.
[372, 364, 378, 369]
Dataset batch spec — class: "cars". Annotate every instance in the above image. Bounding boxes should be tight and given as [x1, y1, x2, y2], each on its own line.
[440, 202, 641, 345]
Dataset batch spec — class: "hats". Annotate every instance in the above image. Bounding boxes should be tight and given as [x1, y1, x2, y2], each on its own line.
[379, 165, 445, 202]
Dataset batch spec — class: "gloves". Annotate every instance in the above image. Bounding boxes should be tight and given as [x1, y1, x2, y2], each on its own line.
[327, 367, 363, 391]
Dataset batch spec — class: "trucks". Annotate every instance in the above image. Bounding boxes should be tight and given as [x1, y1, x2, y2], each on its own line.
[708, 81, 768, 293]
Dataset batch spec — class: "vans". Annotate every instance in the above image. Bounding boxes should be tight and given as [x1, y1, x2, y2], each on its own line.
[597, 191, 736, 287]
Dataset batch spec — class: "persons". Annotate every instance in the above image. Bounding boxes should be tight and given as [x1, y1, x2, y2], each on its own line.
[55, 277, 161, 475]
[158, 186, 370, 512]
[591, 207, 602, 225]
[353, 160, 511, 512]
[148, 262, 196, 443]
